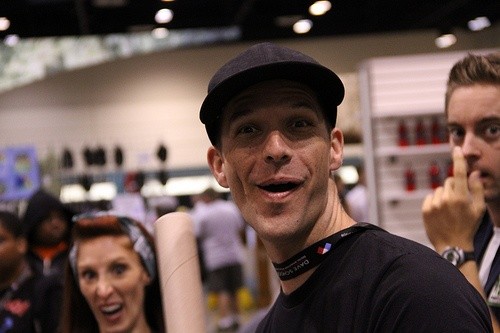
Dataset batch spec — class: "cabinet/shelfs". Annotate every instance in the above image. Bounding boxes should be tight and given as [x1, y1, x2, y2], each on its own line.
[356, 52, 475, 254]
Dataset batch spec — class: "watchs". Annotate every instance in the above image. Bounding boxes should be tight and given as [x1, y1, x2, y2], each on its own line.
[438, 247, 478, 269]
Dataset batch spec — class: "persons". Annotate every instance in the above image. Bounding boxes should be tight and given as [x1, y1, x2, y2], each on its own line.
[344, 161, 370, 222]
[201, 44, 495, 333]
[112, 168, 156, 236]
[66, 215, 168, 333]
[419, 49, 500, 333]
[193, 185, 249, 333]
[0, 209, 47, 333]
[20, 187, 74, 333]
[330, 171, 345, 214]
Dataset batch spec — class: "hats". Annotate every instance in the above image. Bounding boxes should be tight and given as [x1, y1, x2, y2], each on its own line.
[198, 44, 345, 144]
